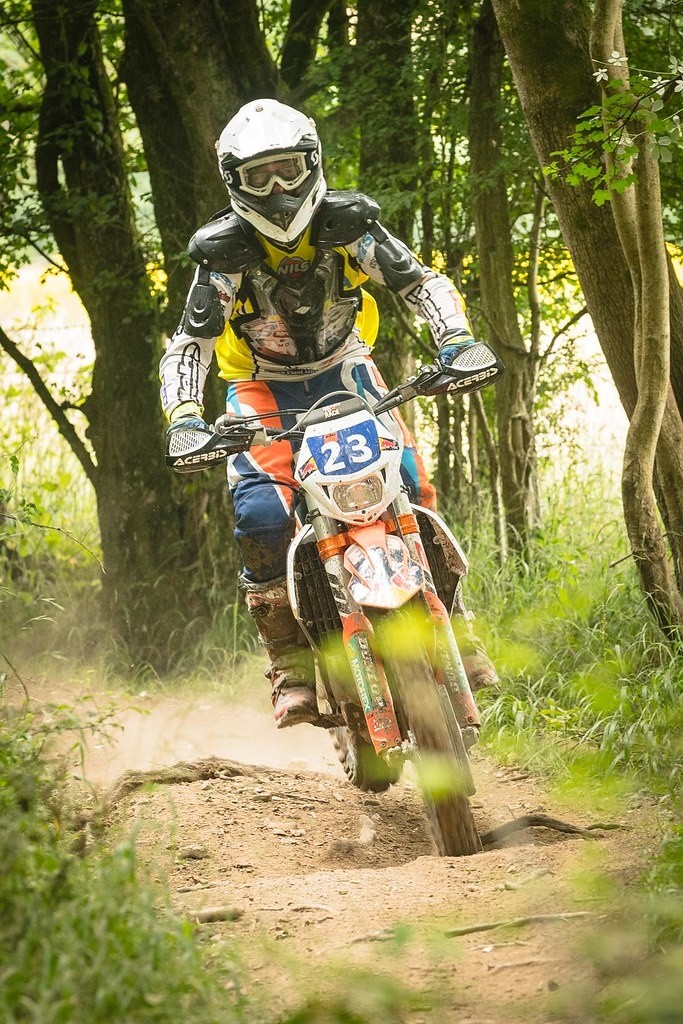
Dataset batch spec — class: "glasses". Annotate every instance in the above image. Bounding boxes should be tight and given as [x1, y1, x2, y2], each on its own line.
[235, 152, 312, 196]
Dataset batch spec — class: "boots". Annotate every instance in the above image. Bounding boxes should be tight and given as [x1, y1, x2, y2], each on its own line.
[449, 580, 499, 692]
[237, 567, 319, 729]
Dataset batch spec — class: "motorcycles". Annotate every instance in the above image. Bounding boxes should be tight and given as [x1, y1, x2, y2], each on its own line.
[163, 339, 504, 859]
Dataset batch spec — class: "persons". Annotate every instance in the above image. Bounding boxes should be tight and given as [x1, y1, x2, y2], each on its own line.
[159, 98, 504, 727]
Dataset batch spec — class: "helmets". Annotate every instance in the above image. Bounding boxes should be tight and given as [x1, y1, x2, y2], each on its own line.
[216, 99, 327, 243]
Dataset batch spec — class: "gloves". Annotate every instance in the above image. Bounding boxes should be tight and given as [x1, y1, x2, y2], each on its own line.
[165, 416, 208, 437]
[438, 339, 477, 366]
